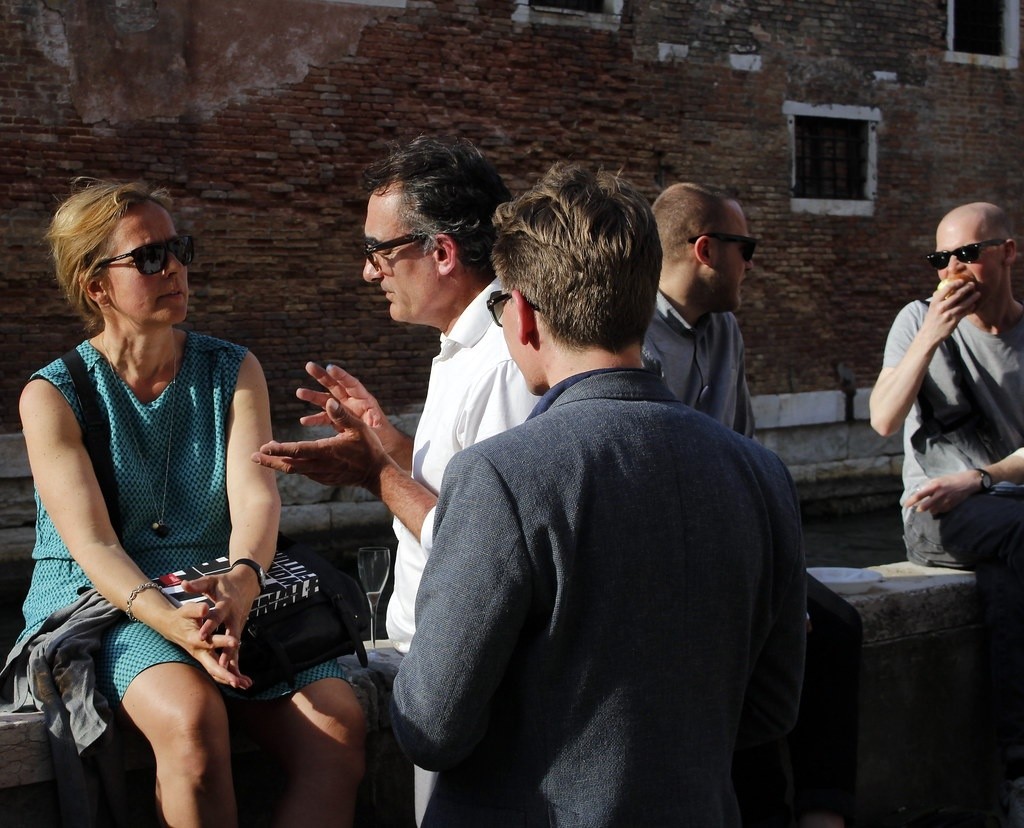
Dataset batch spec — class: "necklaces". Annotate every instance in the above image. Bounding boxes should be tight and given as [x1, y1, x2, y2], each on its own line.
[105, 340, 176, 538]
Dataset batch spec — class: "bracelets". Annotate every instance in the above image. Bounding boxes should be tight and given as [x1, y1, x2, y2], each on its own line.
[126, 582, 164, 623]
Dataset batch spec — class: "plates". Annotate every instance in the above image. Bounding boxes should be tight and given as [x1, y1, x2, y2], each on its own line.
[806, 567, 884, 595]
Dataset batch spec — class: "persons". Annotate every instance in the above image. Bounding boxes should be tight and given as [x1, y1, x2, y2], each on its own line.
[642, 181, 862, 827]
[1, 182, 368, 828]
[384, 159, 810, 827]
[252, 133, 542, 827]
[869, 201, 1024, 828]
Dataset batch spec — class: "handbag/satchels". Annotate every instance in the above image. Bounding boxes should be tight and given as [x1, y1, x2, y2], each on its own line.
[151, 543, 370, 700]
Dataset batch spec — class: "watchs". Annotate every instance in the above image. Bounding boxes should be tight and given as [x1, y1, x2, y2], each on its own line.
[976, 469, 991, 492]
[231, 558, 266, 595]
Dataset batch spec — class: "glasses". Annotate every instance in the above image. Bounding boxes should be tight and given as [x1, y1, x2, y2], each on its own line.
[486, 290, 540, 327]
[926, 239, 1006, 270]
[363, 230, 425, 271]
[688, 232, 758, 262]
[96, 233, 195, 275]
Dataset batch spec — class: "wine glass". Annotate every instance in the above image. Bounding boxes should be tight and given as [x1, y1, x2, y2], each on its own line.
[357, 546, 390, 657]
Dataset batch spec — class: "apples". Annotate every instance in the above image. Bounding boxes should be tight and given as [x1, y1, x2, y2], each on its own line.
[937, 274, 976, 300]
